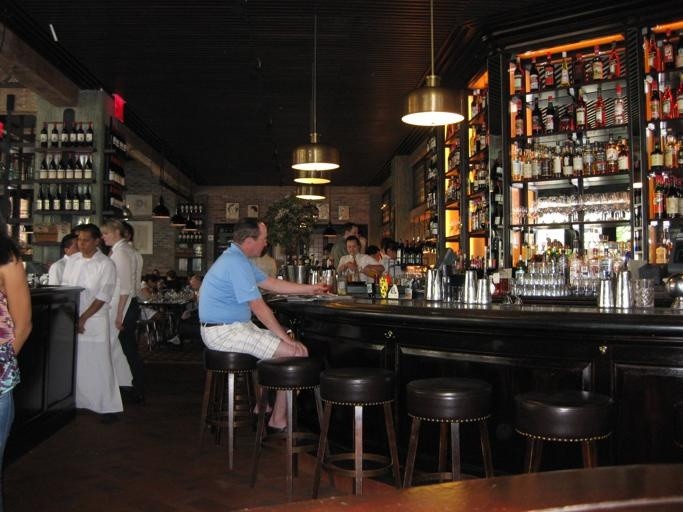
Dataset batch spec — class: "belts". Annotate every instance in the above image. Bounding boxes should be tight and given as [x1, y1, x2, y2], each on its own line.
[199, 322, 234, 326]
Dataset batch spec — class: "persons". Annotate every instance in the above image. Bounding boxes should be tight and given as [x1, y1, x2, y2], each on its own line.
[0, 227, 35, 466]
[49, 218, 202, 415]
[255, 242, 278, 296]
[323, 222, 402, 285]
[197, 218, 330, 434]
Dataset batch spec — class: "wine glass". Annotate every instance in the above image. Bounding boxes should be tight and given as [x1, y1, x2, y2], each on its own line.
[515, 190, 631, 224]
[509, 261, 603, 297]
[147, 285, 194, 304]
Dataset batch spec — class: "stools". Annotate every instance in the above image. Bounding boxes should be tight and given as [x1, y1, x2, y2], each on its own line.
[196, 348, 267, 474]
[513, 388, 617, 472]
[248, 355, 335, 501]
[311, 367, 403, 499]
[403, 377, 495, 489]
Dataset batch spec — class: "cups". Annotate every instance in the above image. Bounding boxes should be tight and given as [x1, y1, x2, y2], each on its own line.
[614, 269, 634, 308]
[631, 279, 655, 308]
[425, 269, 491, 304]
[597, 278, 614, 308]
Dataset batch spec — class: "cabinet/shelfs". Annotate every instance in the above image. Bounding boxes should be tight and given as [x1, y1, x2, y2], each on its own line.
[14, 286, 86, 428]
[0, 105, 129, 279]
[407, 49, 495, 269]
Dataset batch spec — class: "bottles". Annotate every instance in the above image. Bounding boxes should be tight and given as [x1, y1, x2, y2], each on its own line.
[176, 200, 203, 244]
[575, 38, 621, 84]
[513, 234, 634, 277]
[576, 79, 625, 130]
[511, 125, 628, 181]
[0, 121, 127, 211]
[514, 51, 570, 94]
[510, 96, 574, 130]
[396, 61, 505, 273]
[281, 253, 337, 293]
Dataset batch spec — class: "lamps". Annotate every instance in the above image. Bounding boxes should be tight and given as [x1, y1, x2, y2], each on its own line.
[290, 0, 340, 202]
[151, 160, 198, 231]
[402, 0, 465, 126]
